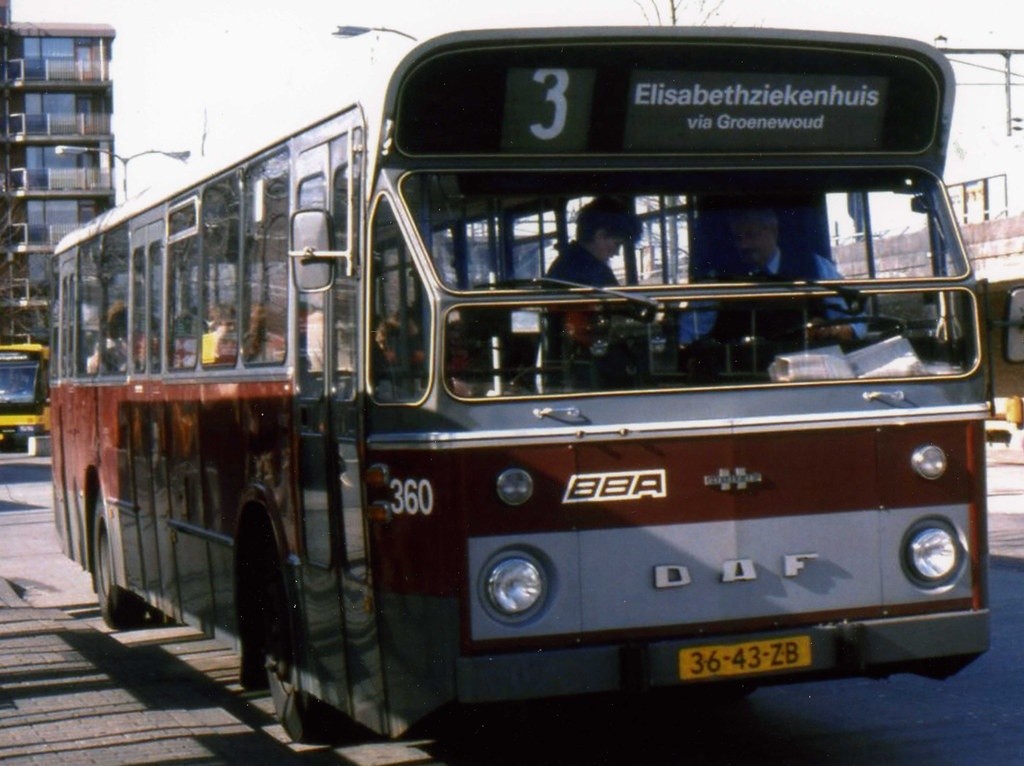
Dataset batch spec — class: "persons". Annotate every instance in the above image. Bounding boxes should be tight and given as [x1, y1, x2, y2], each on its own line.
[680, 202, 869, 346]
[375, 314, 422, 365]
[544, 195, 643, 285]
[87, 302, 196, 367]
[200, 300, 286, 363]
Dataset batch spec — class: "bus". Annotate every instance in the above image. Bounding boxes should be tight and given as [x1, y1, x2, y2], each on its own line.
[50, 26, 990, 741]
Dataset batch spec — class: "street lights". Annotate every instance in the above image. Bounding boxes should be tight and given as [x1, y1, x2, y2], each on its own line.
[56, 146, 191, 203]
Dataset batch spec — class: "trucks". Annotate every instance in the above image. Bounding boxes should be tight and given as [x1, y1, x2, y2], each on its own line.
[0, 343, 51, 449]
